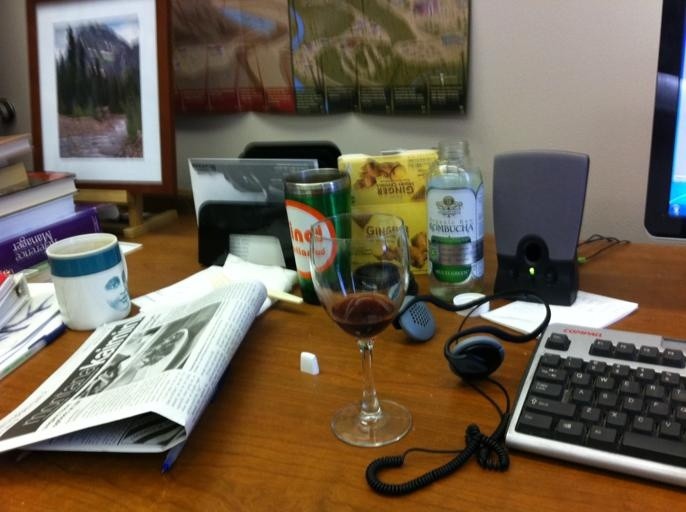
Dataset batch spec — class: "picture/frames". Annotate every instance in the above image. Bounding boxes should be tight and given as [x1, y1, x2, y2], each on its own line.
[25, 0, 179, 198]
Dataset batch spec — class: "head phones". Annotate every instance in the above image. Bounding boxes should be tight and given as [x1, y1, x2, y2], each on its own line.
[390, 286, 550, 376]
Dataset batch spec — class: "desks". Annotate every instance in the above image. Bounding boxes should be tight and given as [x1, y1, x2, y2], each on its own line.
[2, 192, 685, 511]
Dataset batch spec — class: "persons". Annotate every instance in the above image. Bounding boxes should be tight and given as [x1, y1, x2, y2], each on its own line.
[102, 267, 130, 311]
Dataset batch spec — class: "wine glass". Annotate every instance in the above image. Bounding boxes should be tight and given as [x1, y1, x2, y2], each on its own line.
[308, 210, 414, 448]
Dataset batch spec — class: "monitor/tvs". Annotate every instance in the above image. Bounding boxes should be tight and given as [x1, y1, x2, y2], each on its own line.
[644, 0, 686, 242]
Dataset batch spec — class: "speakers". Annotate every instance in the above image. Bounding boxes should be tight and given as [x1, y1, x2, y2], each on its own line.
[492, 150, 591, 306]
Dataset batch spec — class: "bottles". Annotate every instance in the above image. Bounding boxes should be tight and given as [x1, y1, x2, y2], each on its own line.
[423, 136, 488, 303]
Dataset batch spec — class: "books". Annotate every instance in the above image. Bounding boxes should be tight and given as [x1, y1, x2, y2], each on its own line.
[0, 131, 102, 274]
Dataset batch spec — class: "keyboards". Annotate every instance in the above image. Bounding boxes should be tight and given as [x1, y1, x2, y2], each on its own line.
[506, 321, 686, 489]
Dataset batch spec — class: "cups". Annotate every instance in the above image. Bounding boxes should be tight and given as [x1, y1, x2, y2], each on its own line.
[280, 165, 355, 306]
[44, 233, 133, 335]
